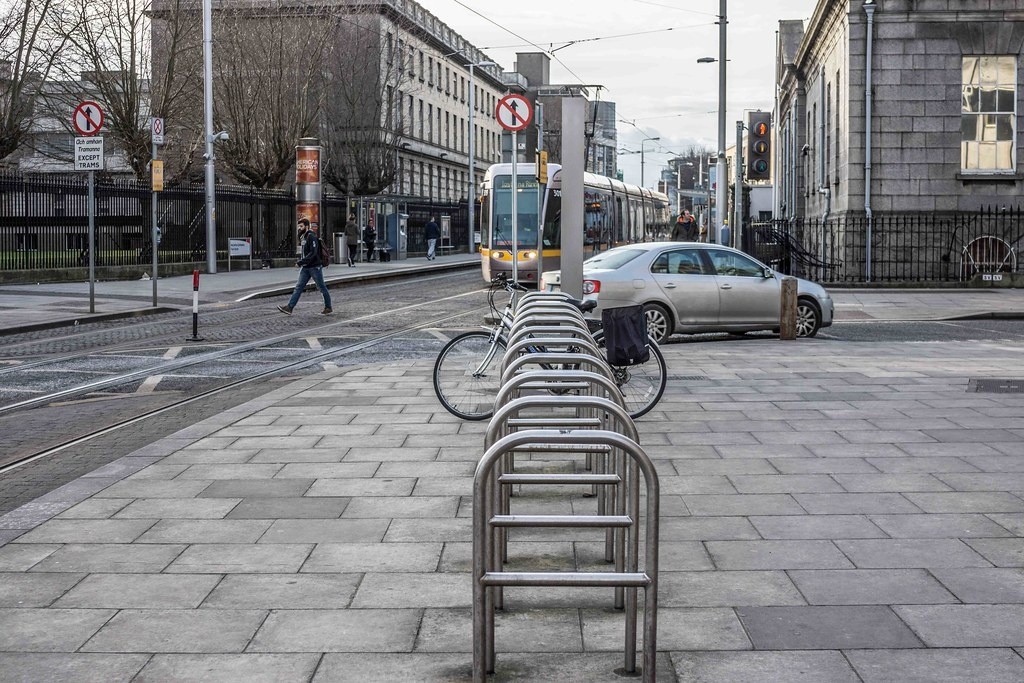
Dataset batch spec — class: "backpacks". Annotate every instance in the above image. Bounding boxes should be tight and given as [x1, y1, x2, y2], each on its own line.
[307, 233, 332, 267]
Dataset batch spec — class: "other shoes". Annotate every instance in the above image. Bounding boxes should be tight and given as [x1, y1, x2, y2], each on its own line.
[426, 255, 431, 260]
[366, 259, 374, 263]
[432, 257, 435, 260]
[351, 265, 355, 267]
[347, 258, 351, 267]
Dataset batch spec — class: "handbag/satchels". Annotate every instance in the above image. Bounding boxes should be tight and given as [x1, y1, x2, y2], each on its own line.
[601, 305, 650, 366]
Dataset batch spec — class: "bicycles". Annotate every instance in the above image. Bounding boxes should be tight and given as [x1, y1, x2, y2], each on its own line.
[433, 271, 667, 421]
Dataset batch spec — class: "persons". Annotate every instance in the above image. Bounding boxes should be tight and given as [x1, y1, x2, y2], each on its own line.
[671, 209, 699, 242]
[425, 216, 440, 261]
[277, 218, 333, 315]
[345, 215, 361, 267]
[364, 220, 377, 262]
[721, 222, 729, 245]
[701, 217, 714, 244]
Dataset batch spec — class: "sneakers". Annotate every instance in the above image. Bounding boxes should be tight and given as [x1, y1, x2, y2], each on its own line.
[320, 306, 333, 315]
[277, 304, 293, 315]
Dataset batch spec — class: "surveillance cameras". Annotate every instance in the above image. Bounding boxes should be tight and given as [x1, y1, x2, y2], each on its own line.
[220, 134, 229, 142]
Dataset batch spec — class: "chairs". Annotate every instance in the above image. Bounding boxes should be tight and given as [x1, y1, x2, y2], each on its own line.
[678, 259, 693, 273]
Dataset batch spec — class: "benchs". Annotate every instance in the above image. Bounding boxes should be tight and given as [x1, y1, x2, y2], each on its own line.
[353, 239, 394, 262]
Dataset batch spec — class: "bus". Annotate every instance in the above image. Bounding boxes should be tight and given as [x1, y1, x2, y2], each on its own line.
[478, 162, 671, 287]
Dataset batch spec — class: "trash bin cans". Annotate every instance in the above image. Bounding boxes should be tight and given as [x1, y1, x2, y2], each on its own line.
[333, 232, 348, 264]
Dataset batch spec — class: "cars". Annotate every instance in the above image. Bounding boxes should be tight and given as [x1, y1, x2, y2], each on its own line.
[541, 241, 835, 339]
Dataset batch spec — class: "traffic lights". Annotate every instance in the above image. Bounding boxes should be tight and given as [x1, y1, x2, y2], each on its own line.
[747, 112, 772, 181]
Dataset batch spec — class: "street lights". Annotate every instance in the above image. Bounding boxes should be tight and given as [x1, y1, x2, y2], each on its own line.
[677, 161, 694, 216]
[640, 137, 661, 187]
[463, 60, 496, 254]
[697, 57, 730, 246]
